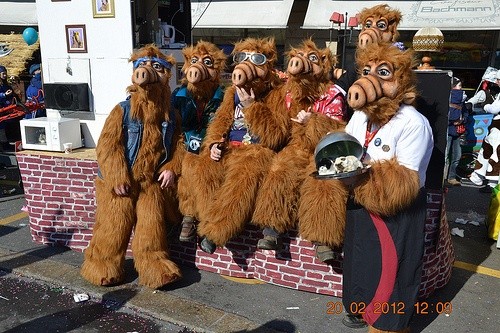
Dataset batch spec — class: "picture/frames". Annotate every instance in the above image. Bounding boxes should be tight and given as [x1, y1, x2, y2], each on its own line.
[65, 24, 87, 53]
[92, 0, 115, 18]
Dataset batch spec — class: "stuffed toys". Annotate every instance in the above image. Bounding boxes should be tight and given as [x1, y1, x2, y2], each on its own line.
[81, 5, 434, 333]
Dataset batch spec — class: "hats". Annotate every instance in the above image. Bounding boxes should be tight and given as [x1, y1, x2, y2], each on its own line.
[453, 77, 460, 87]
[30, 64, 40, 74]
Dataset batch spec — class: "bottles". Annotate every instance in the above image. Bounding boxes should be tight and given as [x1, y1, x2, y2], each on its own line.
[149, 18, 165, 48]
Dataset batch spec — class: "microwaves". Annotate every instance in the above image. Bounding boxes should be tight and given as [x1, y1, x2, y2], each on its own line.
[20, 117, 83, 151]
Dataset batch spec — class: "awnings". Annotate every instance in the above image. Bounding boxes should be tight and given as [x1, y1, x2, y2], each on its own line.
[192, 0, 500, 30]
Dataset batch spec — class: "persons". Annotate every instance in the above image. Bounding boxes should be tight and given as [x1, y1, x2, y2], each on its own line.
[442, 70, 500, 187]
[0, 65, 45, 196]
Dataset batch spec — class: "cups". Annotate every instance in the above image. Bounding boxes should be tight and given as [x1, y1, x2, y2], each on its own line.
[63, 143, 72, 154]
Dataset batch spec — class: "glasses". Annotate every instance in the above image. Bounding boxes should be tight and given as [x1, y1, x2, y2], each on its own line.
[233, 52, 269, 65]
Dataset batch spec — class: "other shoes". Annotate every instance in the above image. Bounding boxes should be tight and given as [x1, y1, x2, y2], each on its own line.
[342, 313, 369, 327]
[448, 179, 459, 185]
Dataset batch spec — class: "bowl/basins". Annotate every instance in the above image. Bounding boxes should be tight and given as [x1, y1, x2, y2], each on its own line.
[314, 131, 364, 164]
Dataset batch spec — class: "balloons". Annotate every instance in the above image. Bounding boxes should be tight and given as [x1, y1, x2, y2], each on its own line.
[22, 28, 37, 45]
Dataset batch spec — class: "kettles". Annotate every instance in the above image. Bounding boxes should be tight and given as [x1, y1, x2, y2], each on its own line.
[162, 24, 175, 44]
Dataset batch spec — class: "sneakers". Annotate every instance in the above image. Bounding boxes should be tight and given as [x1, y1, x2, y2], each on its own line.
[180, 217, 197, 239]
[315, 244, 334, 262]
[200, 236, 214, 253]
[257, 229, 277, 249]
